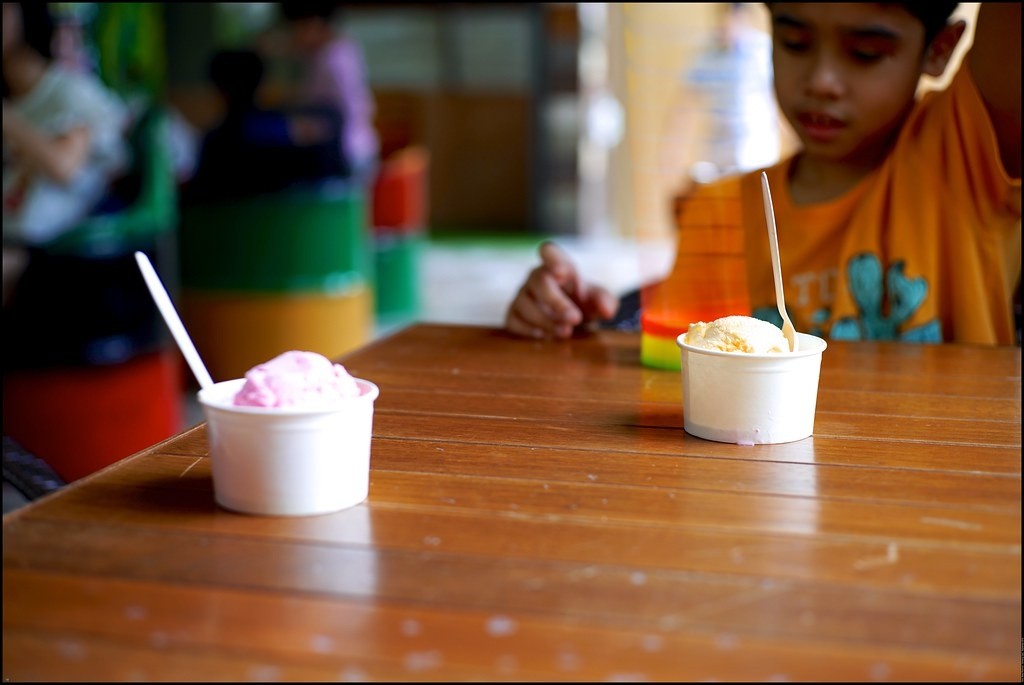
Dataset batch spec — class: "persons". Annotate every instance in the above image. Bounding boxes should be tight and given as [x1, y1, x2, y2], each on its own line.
[504, 0, 1024, 348]
[0, 0, 379, 374]
[681, 0, 779, 184]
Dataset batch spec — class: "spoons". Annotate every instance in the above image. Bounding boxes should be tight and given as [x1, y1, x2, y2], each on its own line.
[761, 172, 799, 353]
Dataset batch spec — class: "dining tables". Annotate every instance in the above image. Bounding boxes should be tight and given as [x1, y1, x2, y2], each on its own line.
[1, 324, 1024, 683]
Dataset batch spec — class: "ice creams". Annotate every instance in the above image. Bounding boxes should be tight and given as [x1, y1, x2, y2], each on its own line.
[230, 350, 367, 410]
[685, 315, 795, 355]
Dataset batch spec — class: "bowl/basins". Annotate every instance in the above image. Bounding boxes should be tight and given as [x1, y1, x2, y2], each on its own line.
[676, 332, 828, 445]
[197, 378, 380, 515]
